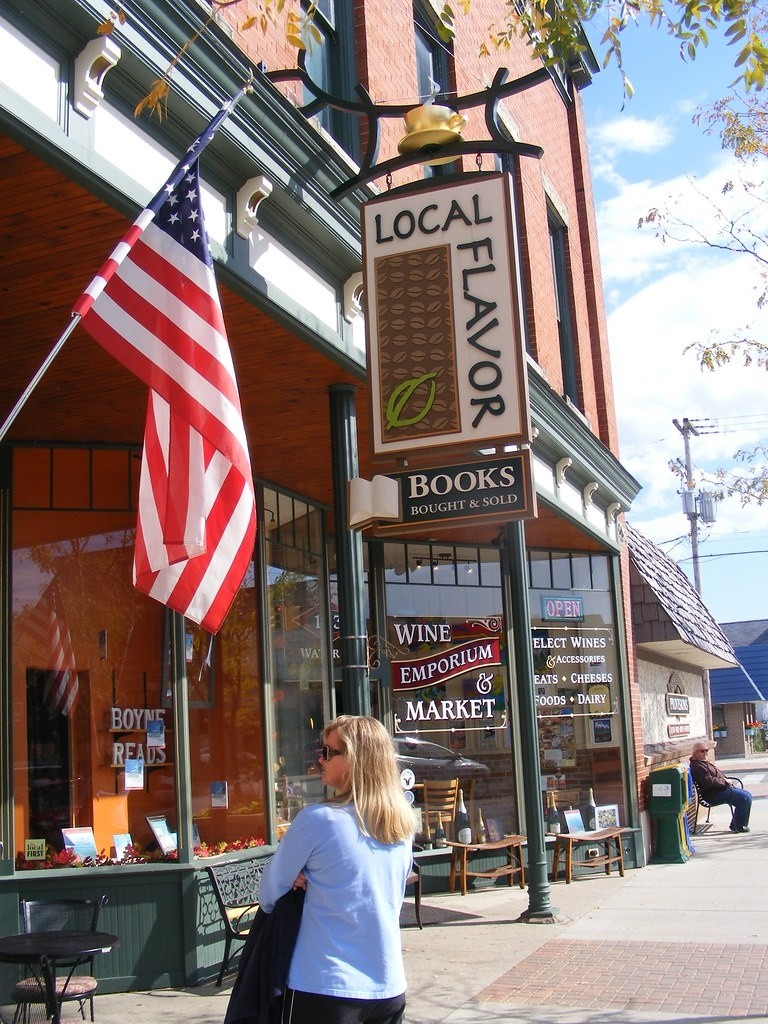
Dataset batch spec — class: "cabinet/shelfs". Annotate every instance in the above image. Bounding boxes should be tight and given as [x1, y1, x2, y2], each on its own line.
[109, 729, 175, 767]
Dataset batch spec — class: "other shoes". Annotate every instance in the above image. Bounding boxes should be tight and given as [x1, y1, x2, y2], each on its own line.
[729, 824, 750, 832]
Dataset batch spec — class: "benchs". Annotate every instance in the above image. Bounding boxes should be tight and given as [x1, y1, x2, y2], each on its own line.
[692, 777, 744, 834]
[438, 836, 527, 895]
[546, 827, 630, 884]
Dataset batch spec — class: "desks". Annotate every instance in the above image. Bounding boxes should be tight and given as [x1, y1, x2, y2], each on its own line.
[545, 788, 582, 808]
[0, 931, 121, 1024]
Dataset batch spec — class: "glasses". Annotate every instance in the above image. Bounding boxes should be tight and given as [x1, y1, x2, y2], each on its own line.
[322, 745, 344, 761]
[697, 749, 709, 753]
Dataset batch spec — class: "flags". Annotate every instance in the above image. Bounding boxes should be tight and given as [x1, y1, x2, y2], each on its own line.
[72, 127, 256, 634]
[48, 581, 81, 716]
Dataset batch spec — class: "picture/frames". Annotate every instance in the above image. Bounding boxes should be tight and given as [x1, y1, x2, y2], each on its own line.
[160, 606, 215, 708]
[146, 814, 177, 855]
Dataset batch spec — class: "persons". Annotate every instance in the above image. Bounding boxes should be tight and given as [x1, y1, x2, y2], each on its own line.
[258, 716, 417, 1024]
[689, 742, 752, 831]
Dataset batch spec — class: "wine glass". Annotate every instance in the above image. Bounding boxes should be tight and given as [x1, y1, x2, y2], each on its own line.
[555, 767, 563, 791]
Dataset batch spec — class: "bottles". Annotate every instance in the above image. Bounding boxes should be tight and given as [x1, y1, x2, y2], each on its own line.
[476, 808, 487, 844]
[569, 805, 572, 810]
[543, 809, 549, 834]
[587, 787, 597, 831]
[424, 826, 433, 849]
[454, 789, 472, 845]
[550, 792, 561, 834]
[435, 812, 446, 848]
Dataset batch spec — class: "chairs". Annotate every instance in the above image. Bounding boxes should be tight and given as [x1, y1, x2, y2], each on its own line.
[421, 777, 459, 842]
[466, 779, 477, 842]
[205, 866, 259, 987]
[10, 894, 109, 1024]
[407, 860, 424, 929]
